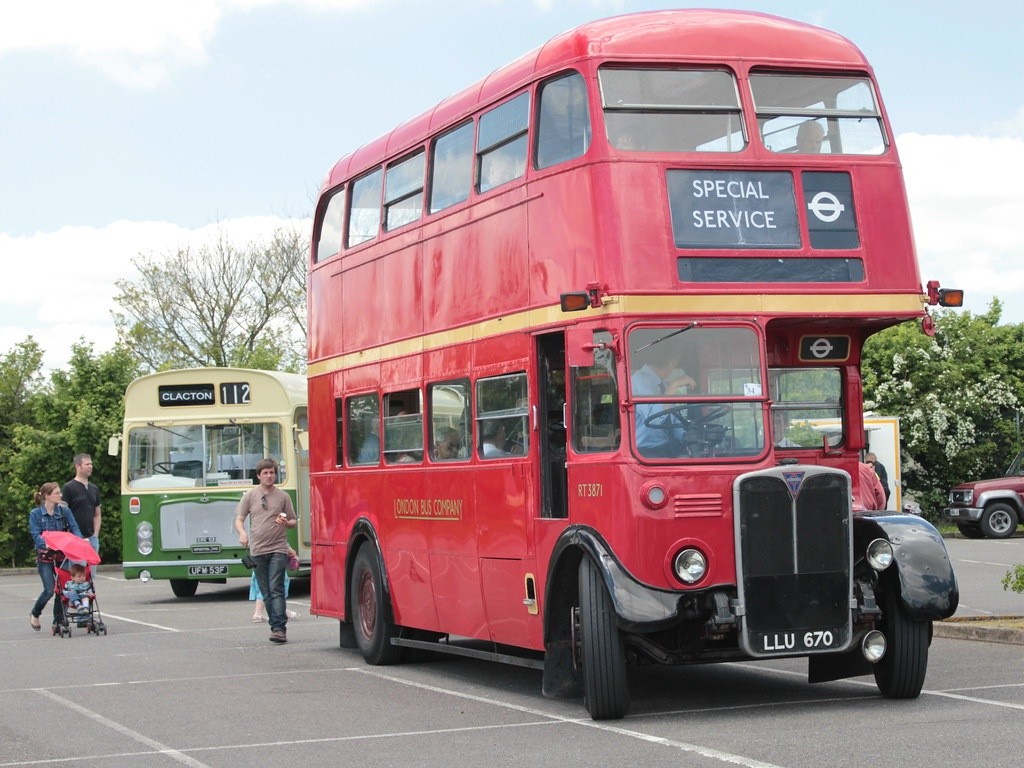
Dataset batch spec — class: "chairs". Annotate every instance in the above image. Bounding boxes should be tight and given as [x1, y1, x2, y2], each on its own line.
[171, 461, 203, 479]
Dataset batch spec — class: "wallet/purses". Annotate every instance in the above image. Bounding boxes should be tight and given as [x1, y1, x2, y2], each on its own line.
[287, 555, 299, 571]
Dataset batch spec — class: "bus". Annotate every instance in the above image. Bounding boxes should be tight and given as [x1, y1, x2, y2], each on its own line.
[108, 366, 311, 599]
[306, 9, 964, 722]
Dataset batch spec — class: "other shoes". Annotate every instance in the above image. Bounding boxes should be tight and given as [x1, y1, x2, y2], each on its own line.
[30, 613, 40, 631]
[77, 604, 89, 614]
[269, 631, 286, 643]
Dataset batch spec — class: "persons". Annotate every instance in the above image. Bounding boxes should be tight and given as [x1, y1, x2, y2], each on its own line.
[234, 459, 302, 643]
[359, 407, 523, 467]
[30, 454, 102, 632]
[750, 412, 802, 447]
[630, 344, 697, 447]
[481, 154, 515, 192]
[859, 451, 891, 511]
[796, 119, 823, 154]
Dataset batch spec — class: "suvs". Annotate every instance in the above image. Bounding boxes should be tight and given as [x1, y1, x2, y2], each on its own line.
[944, 450, 1024, 538]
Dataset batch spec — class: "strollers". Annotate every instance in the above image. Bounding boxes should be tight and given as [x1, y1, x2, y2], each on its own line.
[41, 530, 108, 638]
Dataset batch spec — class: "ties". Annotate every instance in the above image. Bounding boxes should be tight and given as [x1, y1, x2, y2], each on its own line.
[659, 381, 674, 440]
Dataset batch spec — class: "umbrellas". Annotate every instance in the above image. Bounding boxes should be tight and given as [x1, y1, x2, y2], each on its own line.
[41, 528, 101, 580]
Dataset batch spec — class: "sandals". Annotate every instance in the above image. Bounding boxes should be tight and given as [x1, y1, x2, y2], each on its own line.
[253, 612, 269, 622]
[286, 609, 302, 621]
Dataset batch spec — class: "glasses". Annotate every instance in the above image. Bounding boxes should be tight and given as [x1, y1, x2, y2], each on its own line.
[440, 442, 462, 451]
[261, 495, 268, 510]
